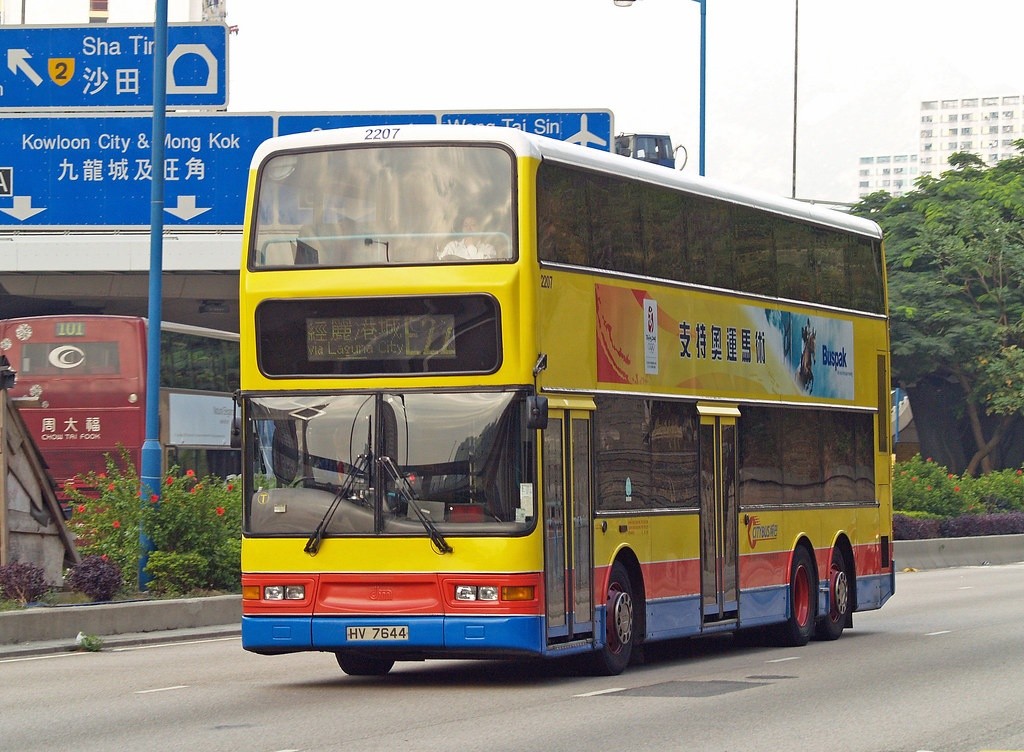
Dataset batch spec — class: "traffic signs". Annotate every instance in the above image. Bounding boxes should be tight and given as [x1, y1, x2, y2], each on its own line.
[0, 107, 616, 234]
[0, 21, 228, 113]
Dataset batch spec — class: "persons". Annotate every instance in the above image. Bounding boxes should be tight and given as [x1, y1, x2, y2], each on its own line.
[437, 216, 498, 264]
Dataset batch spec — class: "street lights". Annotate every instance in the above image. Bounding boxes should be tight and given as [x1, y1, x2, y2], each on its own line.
[613, 0, 707, 176]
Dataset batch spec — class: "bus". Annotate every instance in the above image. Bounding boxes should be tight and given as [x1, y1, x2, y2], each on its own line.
[236, 124, 897, 678]
[1, 314, 240, 553]
